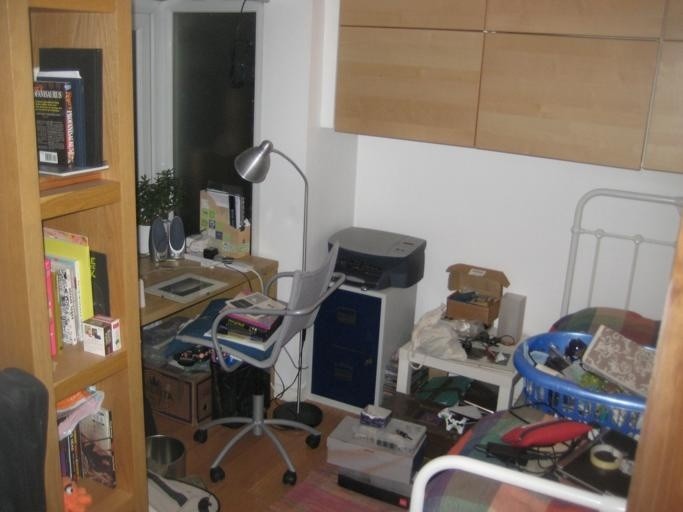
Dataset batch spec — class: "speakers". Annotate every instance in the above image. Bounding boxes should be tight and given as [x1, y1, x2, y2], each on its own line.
[169, 216, 185, 258]
[150, 216, 168, 261]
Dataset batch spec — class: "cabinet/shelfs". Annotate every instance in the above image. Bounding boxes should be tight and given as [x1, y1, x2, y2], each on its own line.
[0, 0, 149, 511]
[334, 1, 683, 173]
[308, 277, 417, 416]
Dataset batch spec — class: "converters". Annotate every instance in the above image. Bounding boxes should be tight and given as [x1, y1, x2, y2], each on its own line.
[489, 440, 526, 465]
[203, 248, 217, 259]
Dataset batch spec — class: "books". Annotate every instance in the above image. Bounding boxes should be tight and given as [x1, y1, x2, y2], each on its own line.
[202, 291, 289, 352]
[33, 48, 117, 491]
[462, 380, 497, 414]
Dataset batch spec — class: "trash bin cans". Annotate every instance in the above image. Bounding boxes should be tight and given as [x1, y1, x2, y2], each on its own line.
[148, 435, 187, 478]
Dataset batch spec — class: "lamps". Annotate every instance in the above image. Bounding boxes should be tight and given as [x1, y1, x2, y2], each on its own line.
[234, 140, 322, 430]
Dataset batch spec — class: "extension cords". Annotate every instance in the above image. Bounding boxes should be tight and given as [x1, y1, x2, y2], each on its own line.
[184, 250, 252, 274]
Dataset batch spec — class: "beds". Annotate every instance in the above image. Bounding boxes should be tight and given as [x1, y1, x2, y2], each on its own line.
[407, 188, 681, 512]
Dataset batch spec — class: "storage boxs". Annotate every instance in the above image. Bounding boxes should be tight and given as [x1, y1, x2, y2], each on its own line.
[326, 416, 427, 485]
[143, 361, 213, 428]
[446, 264, 510, 326]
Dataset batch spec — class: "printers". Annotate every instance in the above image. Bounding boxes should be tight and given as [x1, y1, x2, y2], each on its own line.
[328, 229, 426, 289]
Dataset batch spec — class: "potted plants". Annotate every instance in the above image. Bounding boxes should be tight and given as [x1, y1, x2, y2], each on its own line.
[136, 169, 183, 255]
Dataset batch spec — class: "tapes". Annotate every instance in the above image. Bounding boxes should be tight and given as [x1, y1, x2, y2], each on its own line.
[590, 444, 623, 470]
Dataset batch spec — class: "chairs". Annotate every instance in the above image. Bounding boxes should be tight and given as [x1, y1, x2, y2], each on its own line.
[194, 241, 346, 486]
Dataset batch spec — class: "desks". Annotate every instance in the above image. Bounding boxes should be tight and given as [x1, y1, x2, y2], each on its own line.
[138, 256, 278, 400]
